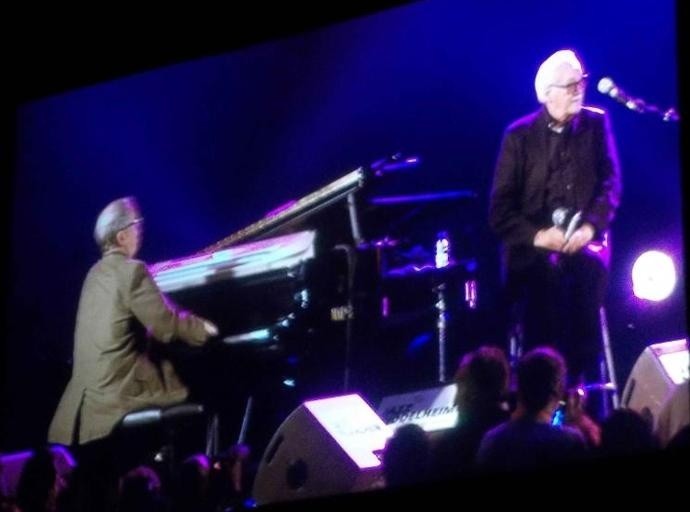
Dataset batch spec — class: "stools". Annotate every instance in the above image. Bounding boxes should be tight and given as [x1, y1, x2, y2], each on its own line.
[121, 401, 206, 470]
[503, 261, 619, 412]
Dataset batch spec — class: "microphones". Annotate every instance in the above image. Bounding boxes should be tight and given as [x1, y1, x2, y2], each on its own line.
[598, 78, 644, 114]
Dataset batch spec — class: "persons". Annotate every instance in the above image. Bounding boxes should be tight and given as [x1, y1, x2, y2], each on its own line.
[489, 49, 621, 378]
[47, 192, 222, 453]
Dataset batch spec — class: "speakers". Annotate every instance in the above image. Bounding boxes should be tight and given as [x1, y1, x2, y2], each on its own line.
[0, 446, 75, 512]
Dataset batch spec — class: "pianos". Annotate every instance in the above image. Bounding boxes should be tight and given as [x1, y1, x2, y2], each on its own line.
[139, 154, 485, 373]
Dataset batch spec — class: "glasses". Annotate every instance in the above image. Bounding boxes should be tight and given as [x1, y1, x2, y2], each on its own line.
[120, 216, 144, 233]
[549, 74, 588, 95]
